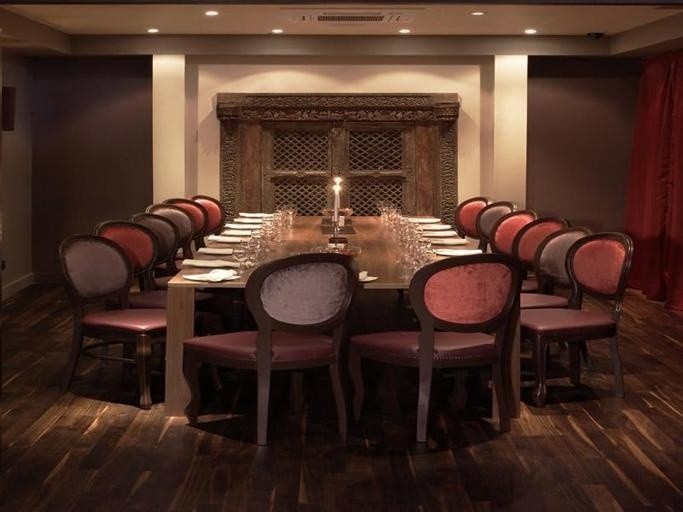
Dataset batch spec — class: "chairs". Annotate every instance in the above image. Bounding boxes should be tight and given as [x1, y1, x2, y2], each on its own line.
[349, 254, 525, 445]
[58, 196, 225, 408]
[181, 253, 356, 446]
[457, 195, 634, 406]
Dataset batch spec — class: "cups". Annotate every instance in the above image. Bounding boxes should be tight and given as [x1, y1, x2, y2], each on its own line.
[359, 271, 368, 280]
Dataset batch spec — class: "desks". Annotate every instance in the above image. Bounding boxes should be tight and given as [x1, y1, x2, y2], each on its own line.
[166, 214, 522, 416]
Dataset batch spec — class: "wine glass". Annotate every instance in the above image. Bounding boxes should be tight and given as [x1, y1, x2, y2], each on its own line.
[232, 203, 297, 275]
[377, 200, 435, 278]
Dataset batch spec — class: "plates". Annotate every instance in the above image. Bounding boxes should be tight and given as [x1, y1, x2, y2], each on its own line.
[358, 276, 379, 282]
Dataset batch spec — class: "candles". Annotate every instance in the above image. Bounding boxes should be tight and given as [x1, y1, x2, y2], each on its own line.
[331, 177, 342, 224]
